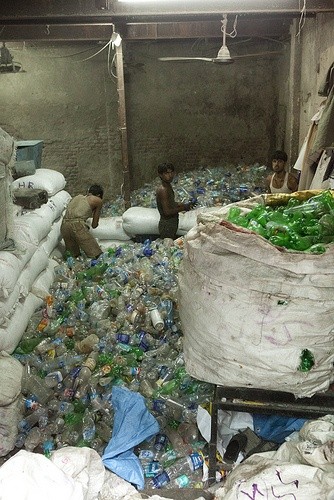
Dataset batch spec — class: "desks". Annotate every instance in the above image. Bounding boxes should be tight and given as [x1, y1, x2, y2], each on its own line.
[208, 385, 334, 487]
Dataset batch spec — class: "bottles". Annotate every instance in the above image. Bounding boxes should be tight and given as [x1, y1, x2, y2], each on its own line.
[96, 163, 272, 216]
[15, 191, 334, 490]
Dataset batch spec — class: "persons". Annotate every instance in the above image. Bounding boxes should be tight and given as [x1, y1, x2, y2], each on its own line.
[266, 150, 298, 193]
[60, 185, 104, 260]
[155, 162, 198, 240]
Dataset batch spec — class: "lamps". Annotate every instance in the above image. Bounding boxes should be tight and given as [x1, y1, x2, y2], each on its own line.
[111, 32, 123, 47]
[0, 42, 28, 74]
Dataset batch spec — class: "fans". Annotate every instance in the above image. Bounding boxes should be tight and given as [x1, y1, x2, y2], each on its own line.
[158, 14, 282, 66]
